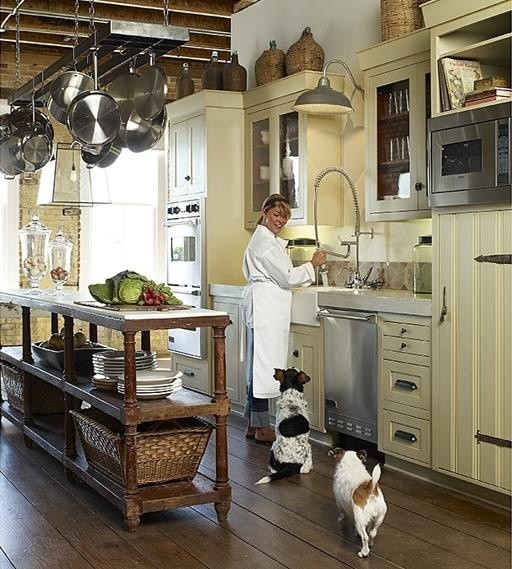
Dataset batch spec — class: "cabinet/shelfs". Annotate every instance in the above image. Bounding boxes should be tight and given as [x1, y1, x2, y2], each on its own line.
[355, 0, 512, 221]
[212, 299, 325, 437]
[161, 89, 245, 397]
[380, 210, 512, 514]
[0, 290, 231, 532]
[242, 70, 346, 226]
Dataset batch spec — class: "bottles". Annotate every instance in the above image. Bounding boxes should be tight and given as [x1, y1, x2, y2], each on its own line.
[411, 235, 432, 295]
[287, 237, 323, 285]
[177, 47, 250, 97]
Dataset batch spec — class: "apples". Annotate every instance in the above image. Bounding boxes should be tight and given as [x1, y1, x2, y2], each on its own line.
[50, 267, 68, 280]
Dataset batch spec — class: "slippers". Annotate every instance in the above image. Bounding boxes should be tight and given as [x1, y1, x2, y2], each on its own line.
[245, 434, 276, 444]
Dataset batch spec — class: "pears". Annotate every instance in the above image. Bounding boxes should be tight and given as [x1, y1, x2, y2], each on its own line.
[39, 327, 94, 353]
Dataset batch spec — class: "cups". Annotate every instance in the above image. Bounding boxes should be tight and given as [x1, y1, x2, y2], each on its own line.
[380, 88, 410, 117]
[385, 136, 410, 162]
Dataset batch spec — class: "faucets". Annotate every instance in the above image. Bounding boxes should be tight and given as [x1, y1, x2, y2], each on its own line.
[311, 165, 375, 289]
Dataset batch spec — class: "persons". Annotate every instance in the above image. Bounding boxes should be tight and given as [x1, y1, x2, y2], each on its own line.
[239, 193, 328, 445]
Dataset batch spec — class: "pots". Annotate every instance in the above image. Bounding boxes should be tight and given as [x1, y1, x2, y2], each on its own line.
[0, 48, 169, 181]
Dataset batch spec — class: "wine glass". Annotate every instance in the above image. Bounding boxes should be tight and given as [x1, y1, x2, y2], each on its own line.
[18, 210, 74, 298]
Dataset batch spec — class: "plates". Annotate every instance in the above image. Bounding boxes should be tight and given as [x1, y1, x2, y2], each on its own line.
[92, 349, 158, 390]
[116, 367, 183, 400]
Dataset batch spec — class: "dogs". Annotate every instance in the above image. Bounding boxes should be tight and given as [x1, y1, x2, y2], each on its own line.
[254, 366, 313, 485]
[328, 447, 387, 558]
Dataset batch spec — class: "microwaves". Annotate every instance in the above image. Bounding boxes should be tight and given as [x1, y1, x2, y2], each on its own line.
[423, 100, 512, 210]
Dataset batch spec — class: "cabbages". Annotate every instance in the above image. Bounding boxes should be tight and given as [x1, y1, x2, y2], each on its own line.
[88, 269, 184, 305]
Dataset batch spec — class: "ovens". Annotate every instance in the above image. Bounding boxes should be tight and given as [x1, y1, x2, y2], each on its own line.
[315, 306, 379, 447]
[163, 196, 208, 362]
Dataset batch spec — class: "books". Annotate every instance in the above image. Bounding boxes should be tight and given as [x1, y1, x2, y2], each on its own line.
[437, 57, 511, 113]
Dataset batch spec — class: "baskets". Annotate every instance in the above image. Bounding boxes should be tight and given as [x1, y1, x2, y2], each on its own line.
[68, 407, 214, 487]
[0, 361, 65, 416]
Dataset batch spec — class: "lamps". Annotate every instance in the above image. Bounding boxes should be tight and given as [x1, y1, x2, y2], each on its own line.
[37, 141, 113, 208]
[294, 60, 365, 114]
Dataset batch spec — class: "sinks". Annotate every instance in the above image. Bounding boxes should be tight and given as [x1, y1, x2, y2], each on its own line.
[320, 283, 361, 292]
